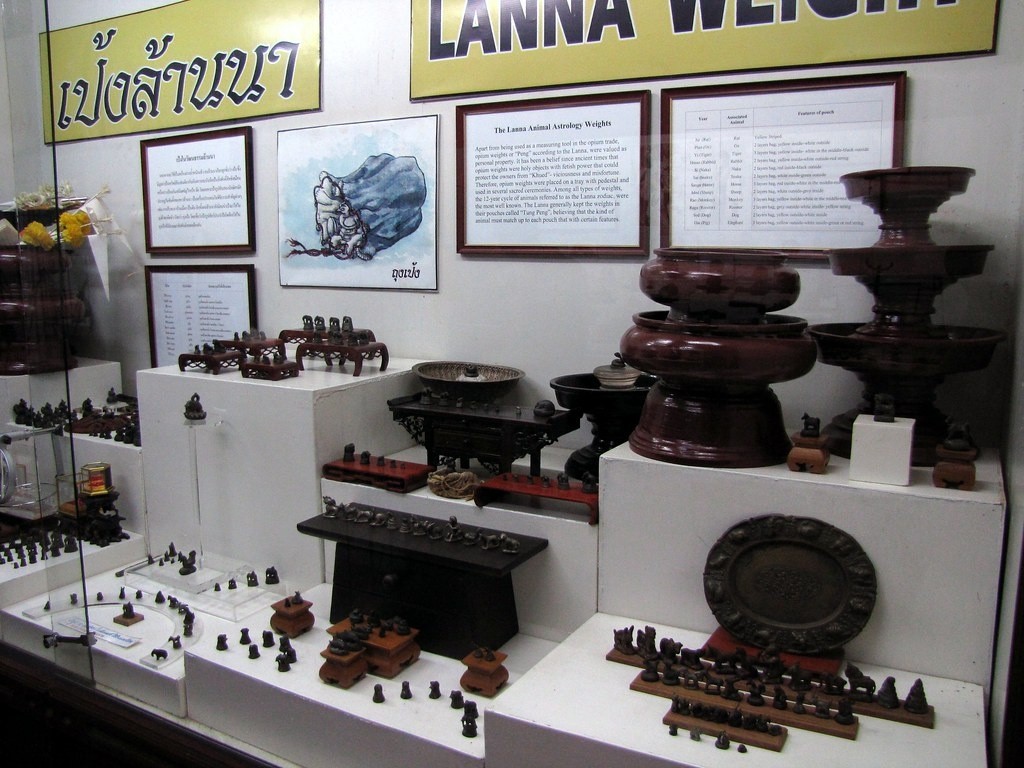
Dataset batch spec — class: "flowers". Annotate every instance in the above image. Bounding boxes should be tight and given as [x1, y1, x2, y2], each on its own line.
[14, 179, 124, 252]
